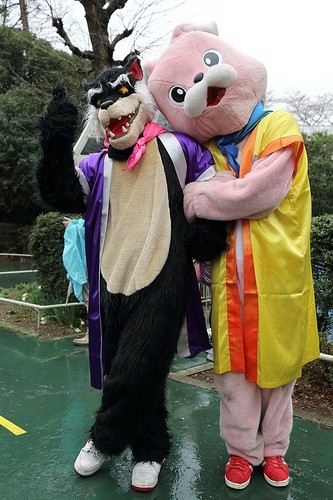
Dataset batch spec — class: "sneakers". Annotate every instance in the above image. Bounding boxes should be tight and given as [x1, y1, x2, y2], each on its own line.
[74, 439, 110, 476]
[224, 454, 253, 489]
[131, 458, 166, 491]
[262, 455, 289, 486]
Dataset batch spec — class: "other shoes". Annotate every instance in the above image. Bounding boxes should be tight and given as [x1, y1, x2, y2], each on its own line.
[73, 335, 89, 345]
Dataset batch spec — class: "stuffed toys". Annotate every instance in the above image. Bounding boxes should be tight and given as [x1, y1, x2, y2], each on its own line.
[35, 57, 225, 491]
[141, 20, 321, 490]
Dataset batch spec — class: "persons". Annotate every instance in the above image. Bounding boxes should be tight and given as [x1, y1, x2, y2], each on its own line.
[62, 216, 89, 345]
